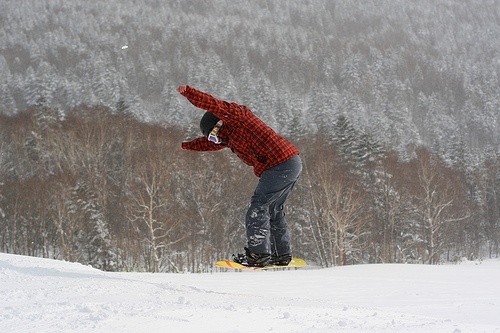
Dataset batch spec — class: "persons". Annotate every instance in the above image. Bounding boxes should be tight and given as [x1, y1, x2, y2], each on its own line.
[176, 84, 303, 268]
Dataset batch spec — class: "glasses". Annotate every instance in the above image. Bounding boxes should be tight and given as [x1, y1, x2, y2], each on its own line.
[207, 126, 220, 145]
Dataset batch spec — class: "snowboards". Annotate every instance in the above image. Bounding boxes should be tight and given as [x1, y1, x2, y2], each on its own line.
[216, 258, 308, 270]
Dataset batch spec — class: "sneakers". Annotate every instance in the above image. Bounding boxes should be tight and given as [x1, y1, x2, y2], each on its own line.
[231, 247, 292, 267]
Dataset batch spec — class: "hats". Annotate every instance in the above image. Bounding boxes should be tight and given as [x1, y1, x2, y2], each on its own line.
[200, 110, 220, 141]
